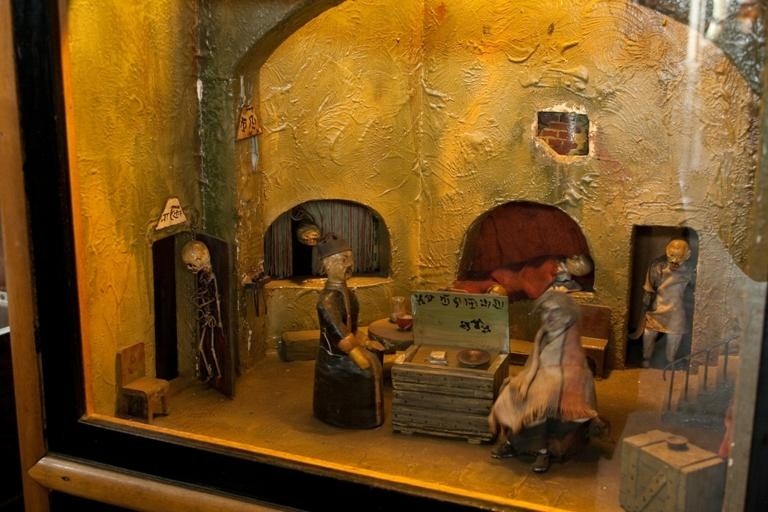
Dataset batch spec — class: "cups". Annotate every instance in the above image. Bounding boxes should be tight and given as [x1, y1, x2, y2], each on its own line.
[389, 296, 406, 323]
[395, 311, 415, 331]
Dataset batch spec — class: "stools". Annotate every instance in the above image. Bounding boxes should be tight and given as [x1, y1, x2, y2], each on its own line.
[312, 345, 385, 429]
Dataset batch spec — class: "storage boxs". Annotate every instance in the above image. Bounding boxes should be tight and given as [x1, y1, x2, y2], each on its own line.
[621, 430, 726, 511]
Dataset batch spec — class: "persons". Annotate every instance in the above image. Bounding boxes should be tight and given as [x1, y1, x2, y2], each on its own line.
[483, 287, 600, 473]
[313, 230, 387, 431]
[441, 253, 591, 300]
[626, 238, 692, 367]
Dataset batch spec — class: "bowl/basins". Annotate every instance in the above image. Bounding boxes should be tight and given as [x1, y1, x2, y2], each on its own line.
[664, 434, 688, 448]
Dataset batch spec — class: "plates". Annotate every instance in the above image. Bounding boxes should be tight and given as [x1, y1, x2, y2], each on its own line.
[456, 348, 491, 366]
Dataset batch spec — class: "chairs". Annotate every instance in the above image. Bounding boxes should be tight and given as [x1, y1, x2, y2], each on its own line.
[116, 342, 171, 424]
[572, 303, 612, 380]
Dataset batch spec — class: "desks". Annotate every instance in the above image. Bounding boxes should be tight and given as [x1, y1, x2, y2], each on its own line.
[392, 343, 511, 447]
[367, 313, 415, 354]
